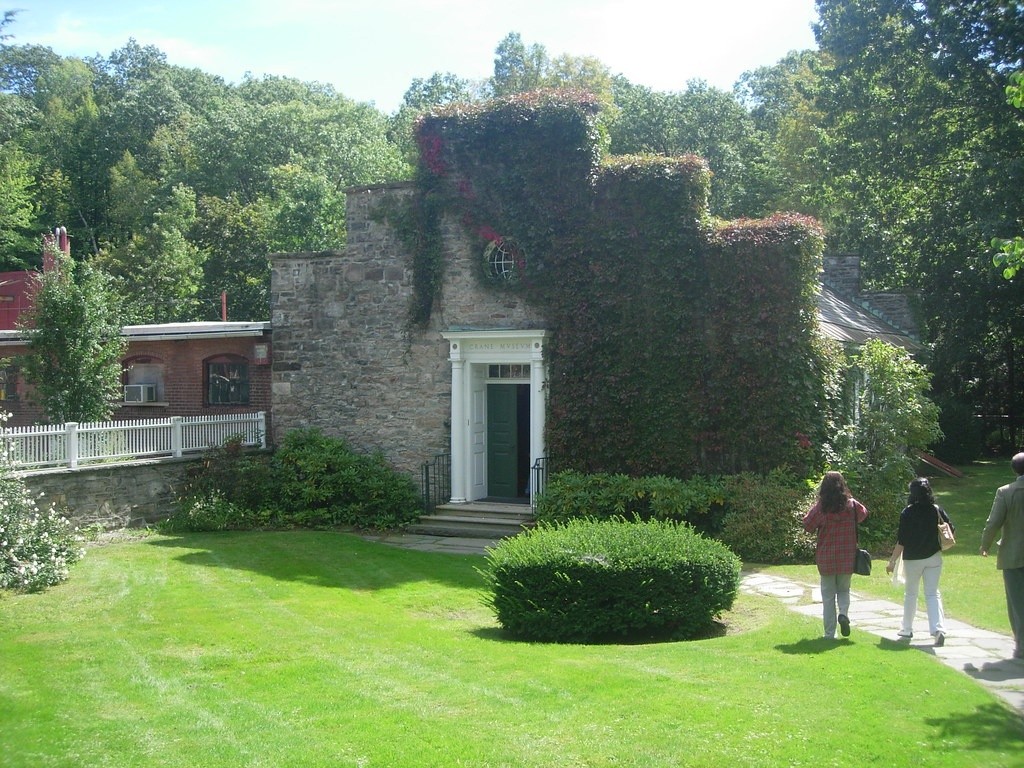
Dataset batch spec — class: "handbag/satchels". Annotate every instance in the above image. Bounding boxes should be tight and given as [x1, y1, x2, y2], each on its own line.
[890, 550, 905, 588]
[933, 503, 956, 551]
[854, 549, 871, 575]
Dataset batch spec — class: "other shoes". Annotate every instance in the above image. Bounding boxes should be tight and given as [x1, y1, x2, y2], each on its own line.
[825, 633, 837, 639]
[838, 614, 850, 636]
[1012, 649, 1024, 658]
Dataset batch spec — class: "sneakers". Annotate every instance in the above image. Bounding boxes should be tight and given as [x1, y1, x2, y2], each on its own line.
[897, 630, 913, 637]
[934, 632, 945, 646]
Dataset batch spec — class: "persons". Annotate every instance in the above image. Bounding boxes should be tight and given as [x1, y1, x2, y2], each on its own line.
[886, 478, 955, 645]
[803, 469, 868, 637]
[981, 452, 1024, 659]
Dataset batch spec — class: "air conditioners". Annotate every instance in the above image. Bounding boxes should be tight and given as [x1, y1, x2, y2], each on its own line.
[124, 384, 156, 404]
